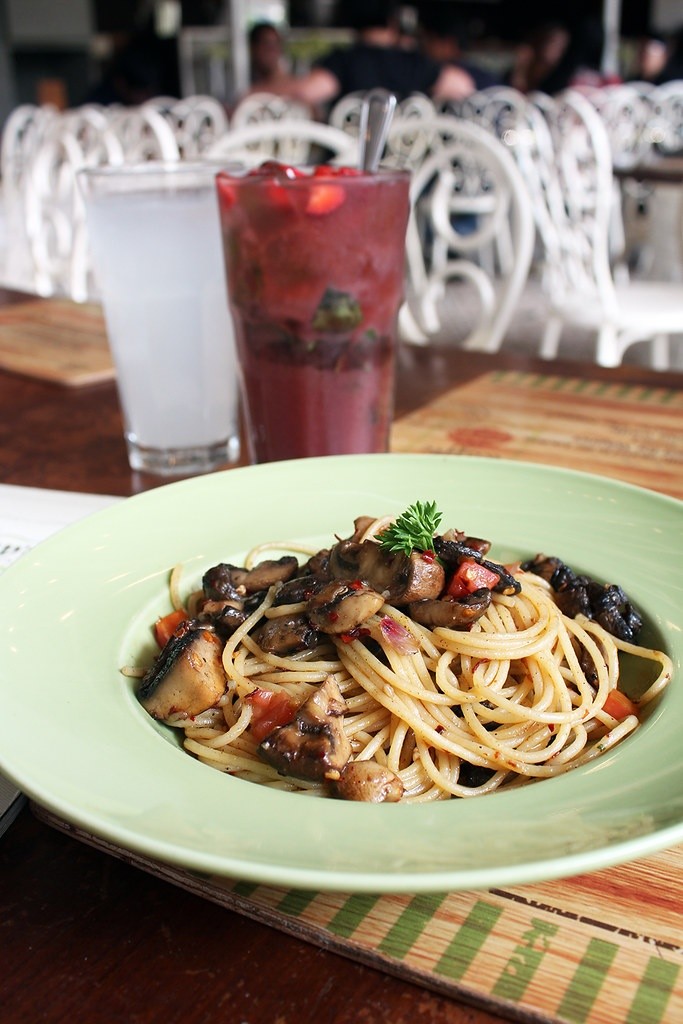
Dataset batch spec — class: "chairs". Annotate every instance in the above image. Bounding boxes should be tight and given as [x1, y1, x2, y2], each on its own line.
[0, 78, 683, 367]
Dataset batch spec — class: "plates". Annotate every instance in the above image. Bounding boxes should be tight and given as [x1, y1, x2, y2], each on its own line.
[1, 453, 683, 894]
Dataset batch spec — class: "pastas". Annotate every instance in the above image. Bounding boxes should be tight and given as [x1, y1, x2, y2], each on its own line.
[168, 542, 671, 802]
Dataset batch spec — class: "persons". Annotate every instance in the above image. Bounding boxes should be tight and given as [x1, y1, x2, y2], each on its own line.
[44, 0, 682, 159]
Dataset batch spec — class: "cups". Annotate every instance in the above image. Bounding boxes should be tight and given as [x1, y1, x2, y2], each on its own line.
[75, 160, 247, 475]
[216, 167, 412, 463]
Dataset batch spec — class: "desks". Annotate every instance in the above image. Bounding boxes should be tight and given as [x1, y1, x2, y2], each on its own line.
[0, 286, 683, 1024]
